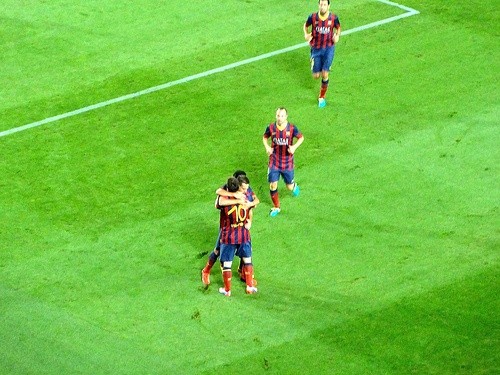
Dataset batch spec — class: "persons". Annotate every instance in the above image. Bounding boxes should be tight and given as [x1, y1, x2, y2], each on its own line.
[263, 108, 304, 216]
[304, 0, 340, 107]
[201, 171, 259, 296]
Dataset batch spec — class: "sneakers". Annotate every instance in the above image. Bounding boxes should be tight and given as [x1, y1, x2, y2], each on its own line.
[241, 273, 257, 286]
[201, 268, 210, 285]
[318, 95, 326, 108]
[218, 287, 231, 298]
[245, 285, 258, 295]
[291, 181, 301, 200]
[269, 208, 280, 217]
